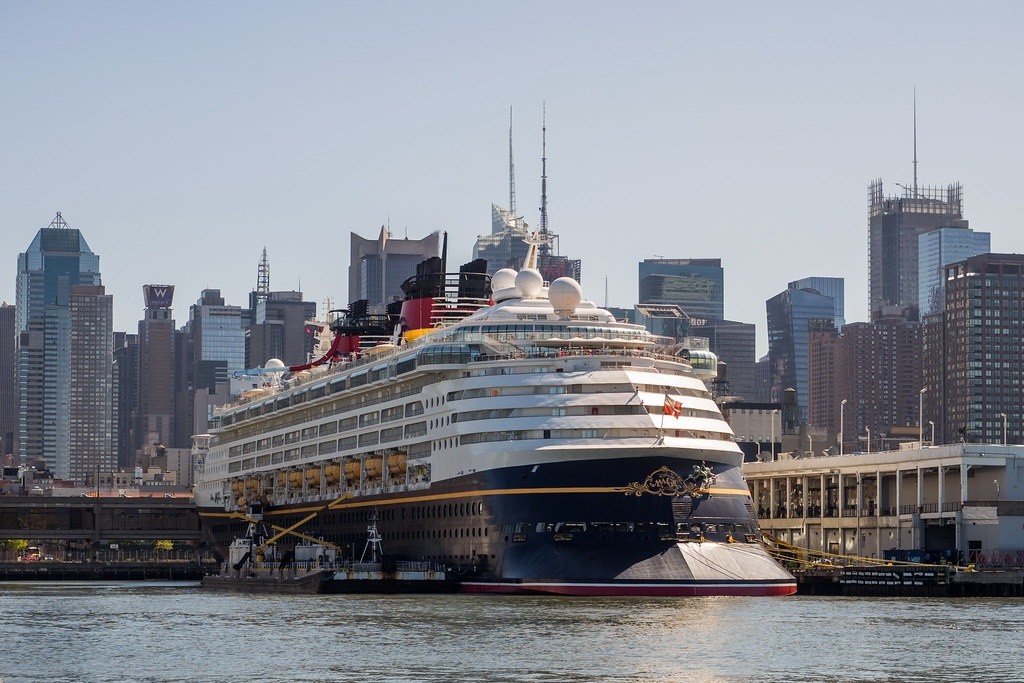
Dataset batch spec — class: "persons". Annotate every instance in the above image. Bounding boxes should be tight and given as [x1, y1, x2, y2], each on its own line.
[960, 559, 966, 567]
[509, 349, 664, 360]
[766, 507, 770, 518]
[940, 557, 946, 565]
[759, 508, 765, 518]
[812, 558, 818, 572]
[776, 504, 821, 517]
[868, 497, 874, 516]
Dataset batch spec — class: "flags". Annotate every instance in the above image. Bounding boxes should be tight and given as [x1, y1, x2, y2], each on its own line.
[662, 395, 682, 419]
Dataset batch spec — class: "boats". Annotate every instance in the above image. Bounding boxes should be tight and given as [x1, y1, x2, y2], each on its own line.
[198, 570, 339, 594]
[190, 230, 801, 599]
[218, 521, 452, 591]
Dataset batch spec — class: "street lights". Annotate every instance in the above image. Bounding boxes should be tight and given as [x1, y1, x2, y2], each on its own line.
[865, 427, 871, 455]
[920, 388, 928, 449]
[840, 398, 848, 456]
[808, 434, 813, 452]
[1001, 412, 1008, 446]
[929, 420, 935, 446]
[755, 441, 761, 454]
[994, 480, 1000, 501]
[772, 409, 777, 462]
[96, 464, 100, 499]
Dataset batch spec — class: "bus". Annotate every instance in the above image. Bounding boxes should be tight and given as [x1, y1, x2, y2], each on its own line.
[17, 549, 25, 561]
[25, 546, 40, 561]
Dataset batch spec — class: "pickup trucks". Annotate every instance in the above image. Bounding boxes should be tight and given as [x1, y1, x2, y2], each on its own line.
[80, 493, 94, 497]
[119, 493, 126, 497]
[164, 494, 173, 499]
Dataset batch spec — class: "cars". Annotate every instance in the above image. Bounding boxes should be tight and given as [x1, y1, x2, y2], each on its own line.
[22, 556, 35, 561]
[39, 551, 42, 559]
[812, 558, 832, 565]
[44, 554, 54, 560]
